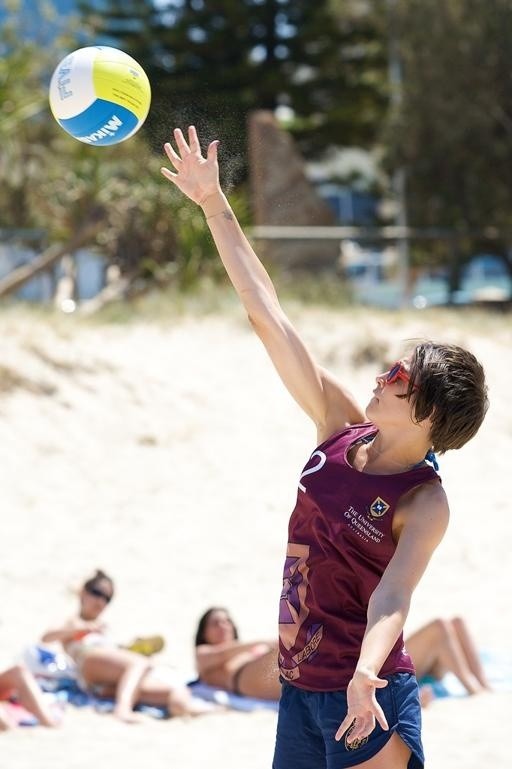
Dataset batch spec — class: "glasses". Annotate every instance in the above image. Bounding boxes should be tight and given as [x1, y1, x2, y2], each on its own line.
[385, 360, 425, 395]
[88, 586, 111, 602]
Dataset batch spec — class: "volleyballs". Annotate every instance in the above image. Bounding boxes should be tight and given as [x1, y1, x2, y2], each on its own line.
[48, 47, 152, 145]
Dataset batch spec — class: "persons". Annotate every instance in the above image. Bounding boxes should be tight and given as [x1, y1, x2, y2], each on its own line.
[404, 616, 492, 695]
[159, 125, 490, 768]
[41, 568, 211, 723]
[0, 665, 55, 733]
[197, 607, 283, 702]
